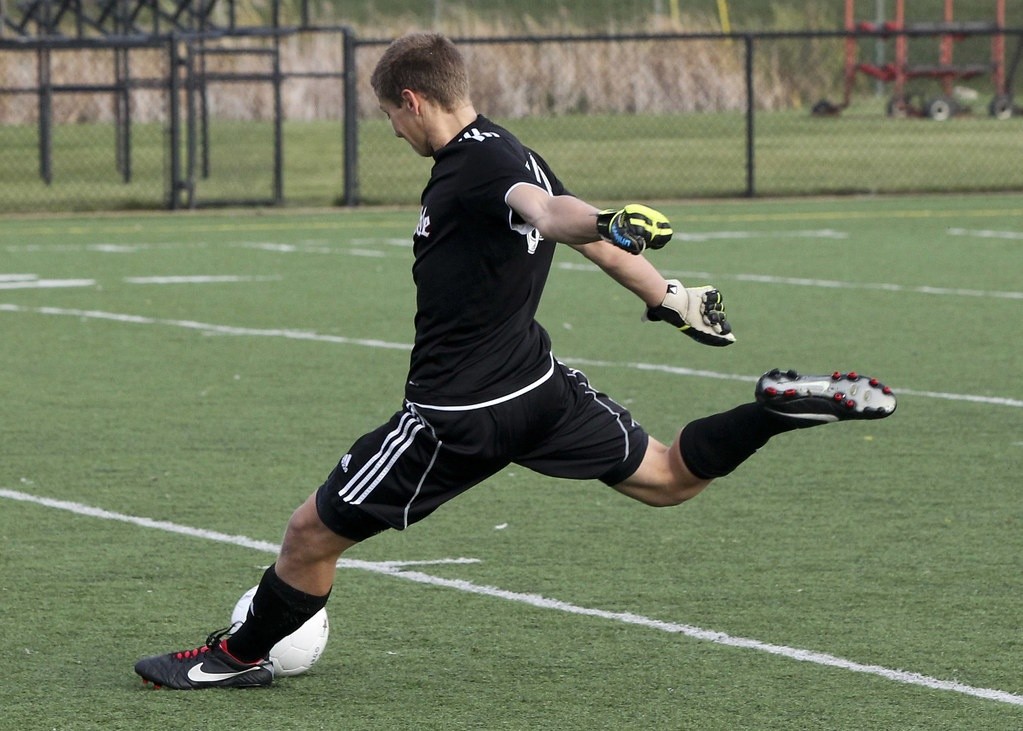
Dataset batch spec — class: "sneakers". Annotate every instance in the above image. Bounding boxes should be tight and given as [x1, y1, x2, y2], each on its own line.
[756, 367, 898, 428]
[135, 621, 275, 690]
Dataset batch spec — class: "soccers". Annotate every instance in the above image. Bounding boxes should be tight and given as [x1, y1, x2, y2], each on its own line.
[230, 583, 331, 678]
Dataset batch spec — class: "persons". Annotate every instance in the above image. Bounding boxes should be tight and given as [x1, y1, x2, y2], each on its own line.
[135, 32, 896, 686]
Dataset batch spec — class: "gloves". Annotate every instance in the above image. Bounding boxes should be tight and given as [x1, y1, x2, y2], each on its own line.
[596, 204, 673, 256]
[646, 278, 737, 347]
